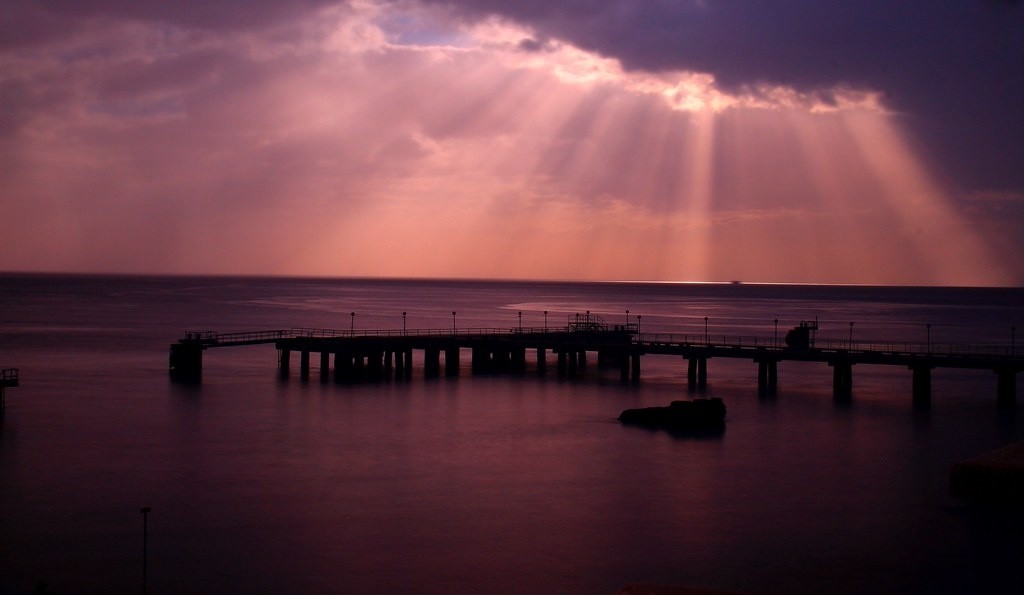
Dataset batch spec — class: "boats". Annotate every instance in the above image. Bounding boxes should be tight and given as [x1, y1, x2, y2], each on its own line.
[794, 320, 818, 330]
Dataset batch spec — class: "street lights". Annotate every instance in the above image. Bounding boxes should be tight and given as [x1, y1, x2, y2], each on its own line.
[587, 311, 590, 332]
[774, 319, 778, 350]
[452, 312, 457, 334]
[544, 311, 547, 332]
[626, 309, 629, 330]
[403, 312, 407, 336]
[927, 324, 931, 353]
[704, 317, 708, 343]
[849, 322, 853, 349]
[637, 315, 641, 341]
[519, 312, 522, 333]
[1011, 326, 1016, 348]
[351, 312, 355, 330]
